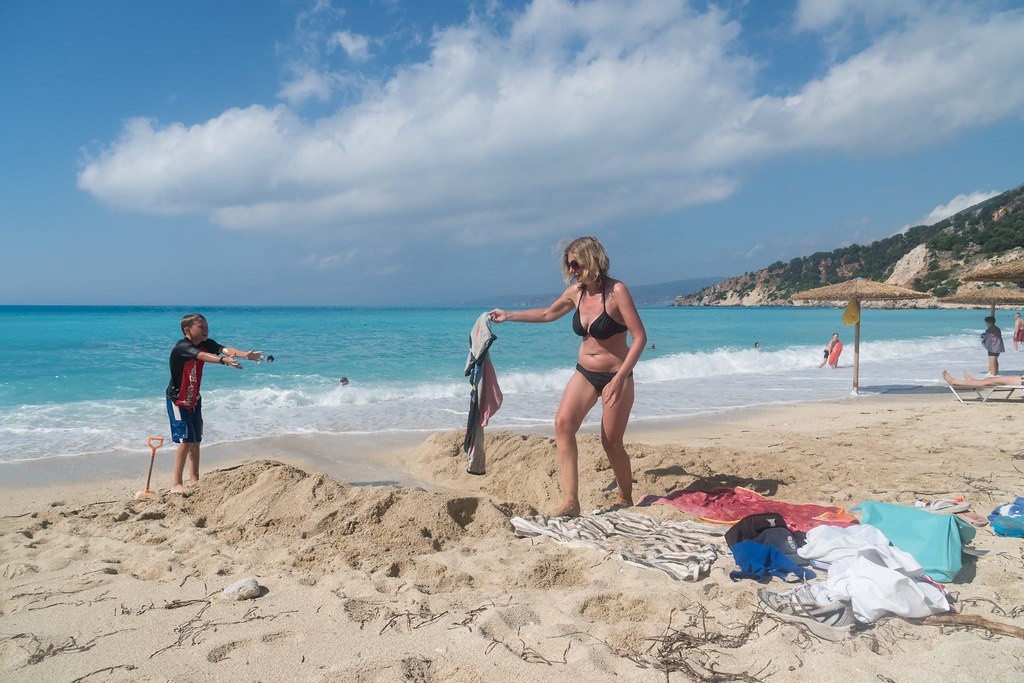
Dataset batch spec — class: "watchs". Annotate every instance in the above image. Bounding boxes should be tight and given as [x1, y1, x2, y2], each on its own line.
[219, 357, 225, 365]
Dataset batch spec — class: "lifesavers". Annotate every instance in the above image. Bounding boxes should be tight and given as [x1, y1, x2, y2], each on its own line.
[827, 342, 843, 367]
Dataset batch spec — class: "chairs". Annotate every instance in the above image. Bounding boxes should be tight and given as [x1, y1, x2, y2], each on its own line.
[948, 384, 1024, 403]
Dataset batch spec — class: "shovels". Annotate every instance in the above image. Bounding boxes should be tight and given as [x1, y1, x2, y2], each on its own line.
[140, 436, 164, 494]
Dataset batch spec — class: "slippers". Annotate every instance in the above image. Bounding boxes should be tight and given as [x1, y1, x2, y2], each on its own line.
[920, 499, 971, 515]
[955, 510, 989, 527]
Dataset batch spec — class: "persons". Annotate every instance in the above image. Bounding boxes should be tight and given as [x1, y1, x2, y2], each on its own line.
[166, 313, 263, 492]
[943, 369, 1024, 389]
[267, 354, 274, 362]
[813, 325, 850, 366]
[1012, 313, 1024, 352]
[483, 237, 647, 518]
[754, 342, 761, 349]
[979, 316, 1005, 378]
[650, 343, 655, 349]
[339, 376, 350, 385]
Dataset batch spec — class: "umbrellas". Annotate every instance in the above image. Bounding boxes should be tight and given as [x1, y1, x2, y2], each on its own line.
[937, 257, 1024, 334]
[788, 274, 931, 395]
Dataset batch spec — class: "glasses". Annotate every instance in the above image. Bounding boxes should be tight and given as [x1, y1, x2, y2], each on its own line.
[568, 260, 580, 271]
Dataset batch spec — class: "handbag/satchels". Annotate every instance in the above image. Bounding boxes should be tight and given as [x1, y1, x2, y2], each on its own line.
[725, 513, 788, 547]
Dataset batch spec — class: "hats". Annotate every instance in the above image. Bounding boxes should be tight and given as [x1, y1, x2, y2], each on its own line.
[755, 528, 808, 565]
